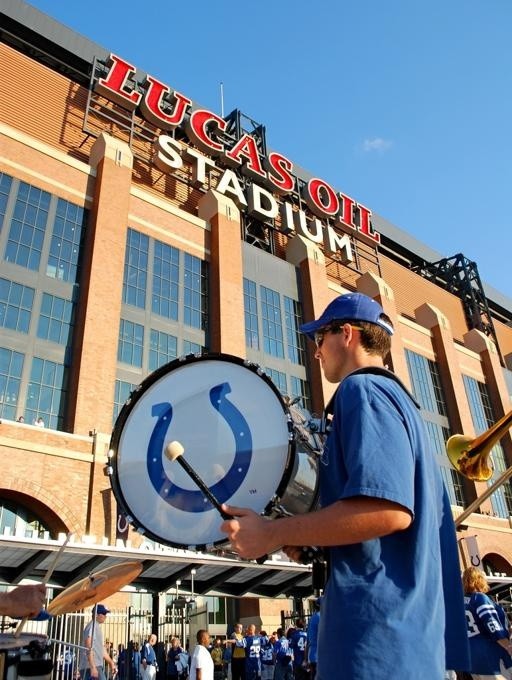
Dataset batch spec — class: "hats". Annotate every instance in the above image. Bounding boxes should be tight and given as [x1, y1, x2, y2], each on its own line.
[298, 292, 394, 342]
[92, 604, 109, 615]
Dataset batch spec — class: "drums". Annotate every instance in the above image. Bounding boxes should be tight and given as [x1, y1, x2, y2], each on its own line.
[103, 354, 324, 559]
[1, 632, 49, 678]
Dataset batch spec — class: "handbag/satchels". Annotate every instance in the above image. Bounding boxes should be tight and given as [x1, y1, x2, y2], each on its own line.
[15, 640, 52, 676]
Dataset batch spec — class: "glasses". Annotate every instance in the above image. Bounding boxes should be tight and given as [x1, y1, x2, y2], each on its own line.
[313, 325, 363, 349]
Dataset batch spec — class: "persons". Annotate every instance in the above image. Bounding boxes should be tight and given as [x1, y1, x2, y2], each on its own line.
[0, 584, 48, 620]
[218, 293, 470, 680]
[454, 566, 510, 680]
[52, 596, 323, 680]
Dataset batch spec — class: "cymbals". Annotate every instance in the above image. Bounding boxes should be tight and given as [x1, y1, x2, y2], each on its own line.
[47, 562, 143, 617]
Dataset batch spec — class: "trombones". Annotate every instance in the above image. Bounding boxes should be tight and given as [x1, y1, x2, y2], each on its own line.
[446, 409, 511, 529]
